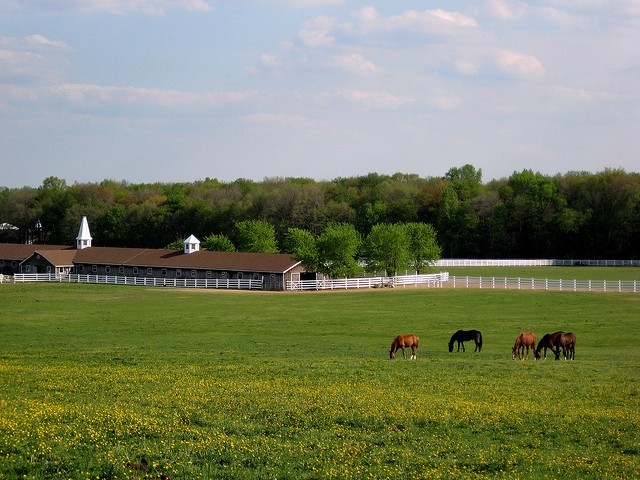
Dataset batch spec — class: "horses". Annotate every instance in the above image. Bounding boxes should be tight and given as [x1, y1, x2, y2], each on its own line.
[389, 334, 420, 361]
[511, 331, 537, 361]
[554, 332, 575, 361]
[534, 331, 568, 363]
[448, 330, 483, 352]
